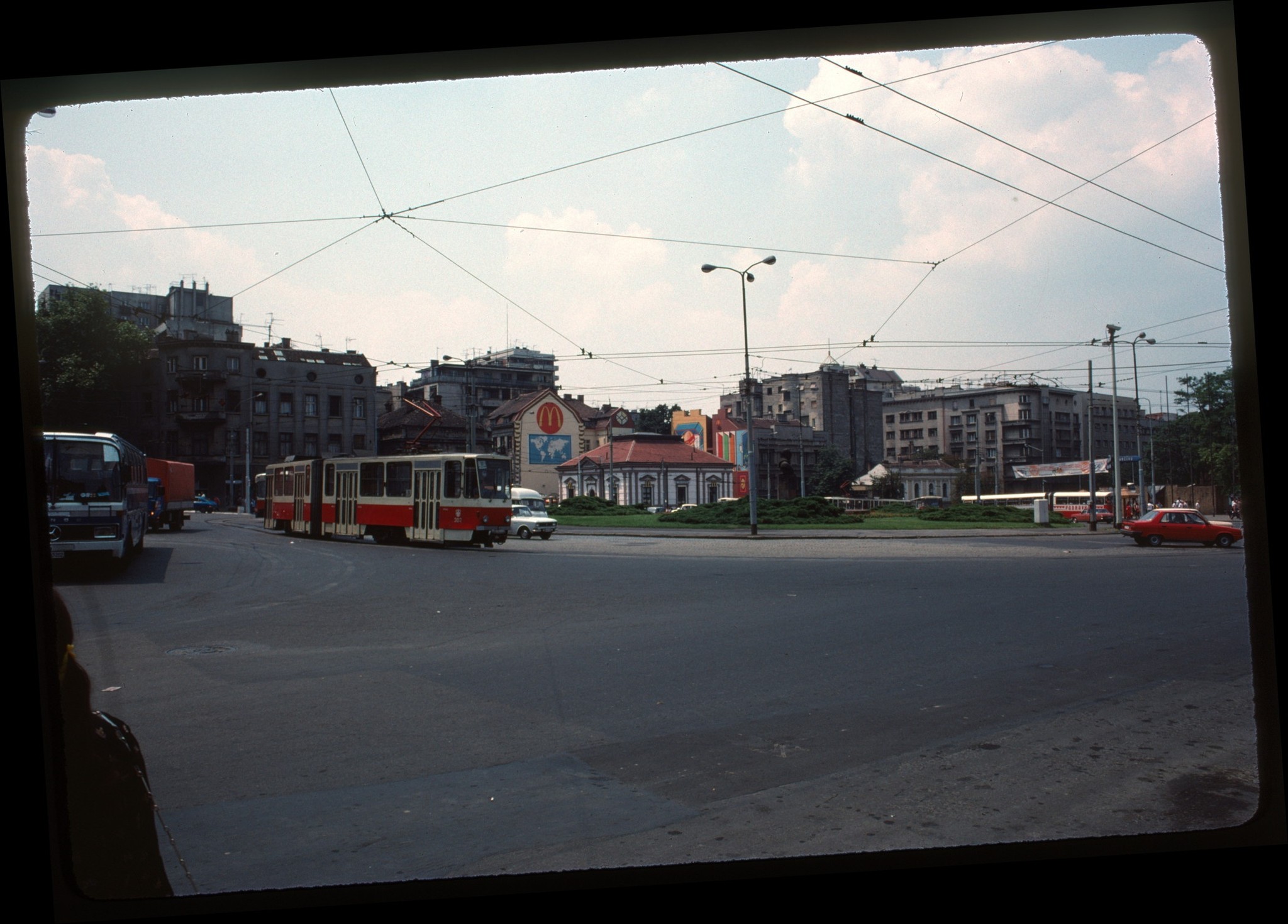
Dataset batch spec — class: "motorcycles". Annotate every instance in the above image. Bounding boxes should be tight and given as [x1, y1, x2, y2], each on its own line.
[1229, 505, 1243, 519]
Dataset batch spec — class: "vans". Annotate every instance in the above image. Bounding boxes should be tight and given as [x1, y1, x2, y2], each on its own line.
[497, 485, 548, 517]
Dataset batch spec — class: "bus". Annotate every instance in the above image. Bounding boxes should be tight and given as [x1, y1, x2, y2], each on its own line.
[43, 431, 148, 566]
[255, 473, 294, 518]
[1053, 488, 1140, 520]
[823, 480, 943, 515]
[264, 396, 514, 548]
[961, 492, 1052, 511]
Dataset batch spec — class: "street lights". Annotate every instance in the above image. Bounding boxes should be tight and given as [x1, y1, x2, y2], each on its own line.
[987, 413, 998, 494]
[230, 393, 263, 512]
[442, 355, 491, 454]
[1106, 324, 1122, 531]
[1101, 331, 1156, 517]
[1024, 443, 1045, 492]
[1133, 397, 1156, 509]
[781, 386, 819, 497]
[701, 255, 777, 532]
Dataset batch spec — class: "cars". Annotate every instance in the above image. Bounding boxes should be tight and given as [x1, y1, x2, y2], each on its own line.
[508, 505, 557, 540]
[193, 496, 217, 514]
[1071, 508, 1114, 524]
[1120, 507, 1244, 549]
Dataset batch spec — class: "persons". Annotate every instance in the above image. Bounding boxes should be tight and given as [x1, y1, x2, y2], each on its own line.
[50, 588, 174, 901]
[1062, 497, 1112, 505]
[197, 491, 256, 514]
[1171, 497, 1188, 523]
[480, 471, 498, 492]
[1126, 500, 1162, 519]
[1196, 500, 1200, 515]
[1231, 497, 1242, 520]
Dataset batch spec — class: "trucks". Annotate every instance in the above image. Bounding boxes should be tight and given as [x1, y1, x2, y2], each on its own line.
[147, 457, 196, 531]
